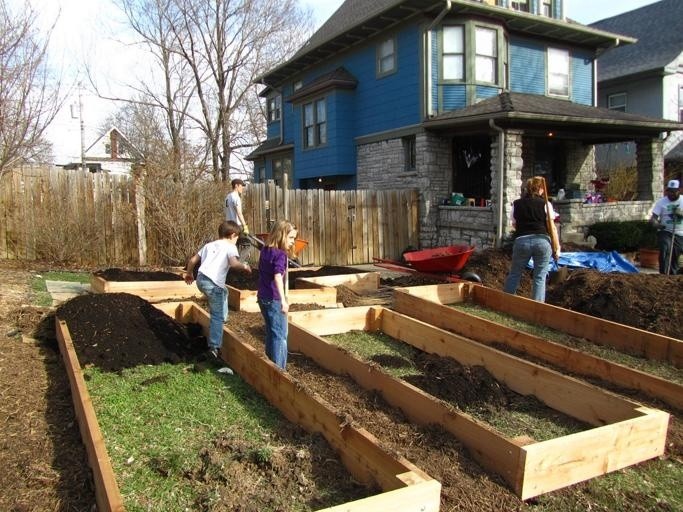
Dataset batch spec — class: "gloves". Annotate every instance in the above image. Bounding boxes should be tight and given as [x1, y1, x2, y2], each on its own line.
[243, 225, 248, 234]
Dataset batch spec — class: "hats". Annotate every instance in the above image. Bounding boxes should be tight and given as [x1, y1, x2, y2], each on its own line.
[232, 179, 245, 186]
[666, 179, 680, 192]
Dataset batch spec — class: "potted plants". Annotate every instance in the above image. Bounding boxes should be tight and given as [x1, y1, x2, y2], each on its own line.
[584, 220, 661, 269]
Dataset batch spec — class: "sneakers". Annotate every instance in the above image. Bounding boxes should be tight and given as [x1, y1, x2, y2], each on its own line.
[208, 348, 225, 366]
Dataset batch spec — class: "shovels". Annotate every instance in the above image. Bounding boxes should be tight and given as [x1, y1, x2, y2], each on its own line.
[542, 177, 567, 286]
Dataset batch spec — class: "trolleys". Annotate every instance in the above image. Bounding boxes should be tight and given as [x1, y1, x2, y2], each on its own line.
[373, 246, 482, 282]
[244, 233, 309, 267]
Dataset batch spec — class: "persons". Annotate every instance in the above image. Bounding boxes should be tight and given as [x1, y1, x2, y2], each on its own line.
[648, 180, 683, 275]
[503, 175, 560, 303]
[225, 179, 252, 263]
[257, 218, 300, 369]
[185, 220, 252, 364]
